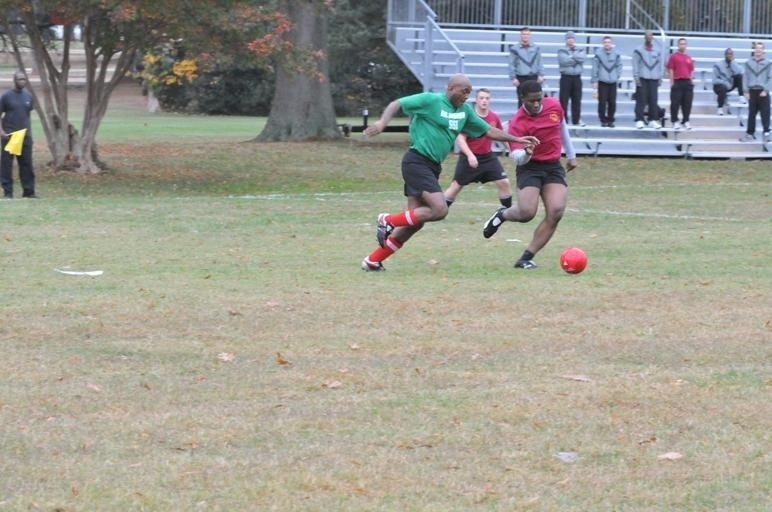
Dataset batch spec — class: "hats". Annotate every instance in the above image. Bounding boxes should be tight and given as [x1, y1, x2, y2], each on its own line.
[565, 31, 575, 38]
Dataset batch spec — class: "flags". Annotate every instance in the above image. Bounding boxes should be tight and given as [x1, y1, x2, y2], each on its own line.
[4, 128, 28, 156]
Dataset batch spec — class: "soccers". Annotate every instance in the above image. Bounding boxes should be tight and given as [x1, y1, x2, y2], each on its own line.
[559, 248, 588, 274]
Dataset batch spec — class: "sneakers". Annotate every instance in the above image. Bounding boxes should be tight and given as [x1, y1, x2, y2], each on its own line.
[648, 120, 661, 129]
[361, 255, 386, 272]
[684, 122, 691, 129]
[376, 213, 394, 248]
[673, 121, 680, 128]
[763, 132, 771, 142]
[484, 206, 507, 238]
[739, 135, 753, 142]
[578, 119, 585, 126]
[515, 259, 538, 269]
[739, 96, 747, 104]
[602, 121, 608, 126]
[608, 121, 615, 127]
[637, 121, 644, 128]
[717, 107, 724, 116]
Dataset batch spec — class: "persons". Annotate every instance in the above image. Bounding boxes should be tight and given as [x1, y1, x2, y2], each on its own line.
[0, 71, 38, 199]
[632, 30, 663, 129]
[483, 80, 577, 270]
[667, 37, 694, 130]
[441, 86, 515, 211]
[508, 26, 544, 110]
[558, 30, 587, 128]
[591, 36, 623, 128]
[711, 48, 747, 115]
[359, 73, 542, 272]
[740, 42, 772, 141]
